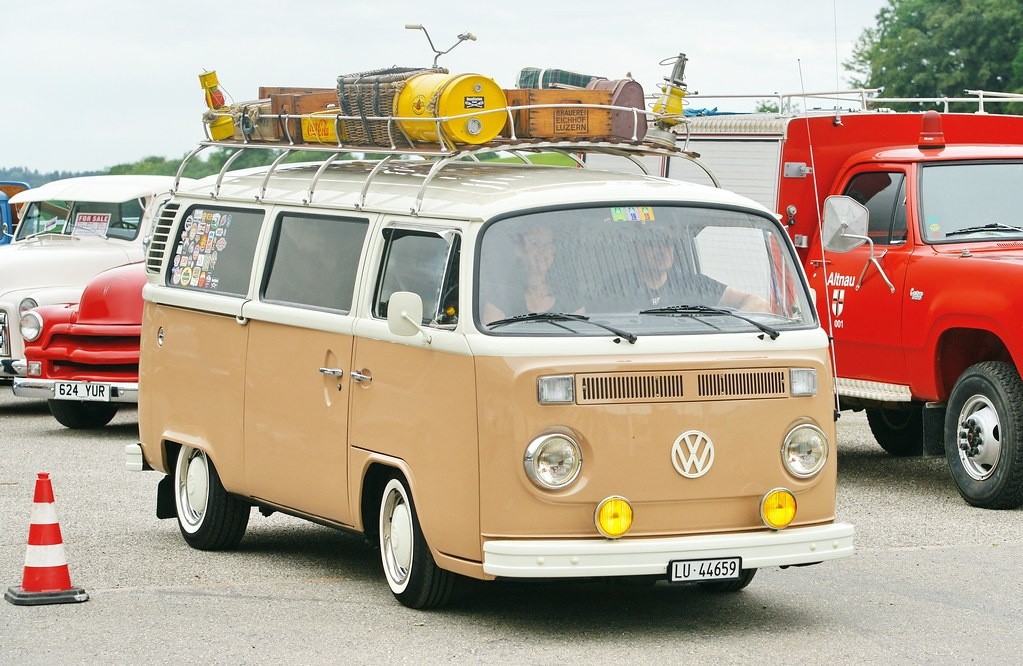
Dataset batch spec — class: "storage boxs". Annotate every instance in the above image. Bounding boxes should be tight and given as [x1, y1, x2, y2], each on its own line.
[499, 89, 614, 138]
[271, 91, 339, 142]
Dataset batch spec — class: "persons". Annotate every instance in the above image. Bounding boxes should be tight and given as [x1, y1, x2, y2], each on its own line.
[479, 222, 771, 324]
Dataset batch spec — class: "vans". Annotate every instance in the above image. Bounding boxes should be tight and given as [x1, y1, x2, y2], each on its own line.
[138, 148, 855, 612]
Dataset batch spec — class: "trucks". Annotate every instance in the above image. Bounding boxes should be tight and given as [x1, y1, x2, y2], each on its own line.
[582, 88, 1023, 511]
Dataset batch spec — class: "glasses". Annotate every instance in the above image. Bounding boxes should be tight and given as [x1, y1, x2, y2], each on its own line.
[646, 240, 674, 247]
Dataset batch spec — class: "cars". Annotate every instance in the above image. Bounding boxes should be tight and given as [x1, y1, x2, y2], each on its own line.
[11, 243, 147, 429]
[0, 174, 198, 382]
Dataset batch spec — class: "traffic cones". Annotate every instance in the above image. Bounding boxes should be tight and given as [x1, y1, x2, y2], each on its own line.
[5, 472, 89, 606]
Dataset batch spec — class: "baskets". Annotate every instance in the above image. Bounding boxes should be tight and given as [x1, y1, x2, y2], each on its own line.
[336, 64, 449, 147]
